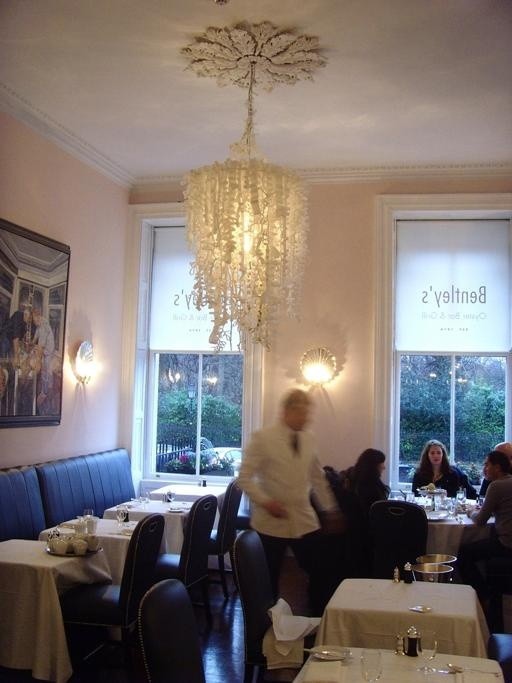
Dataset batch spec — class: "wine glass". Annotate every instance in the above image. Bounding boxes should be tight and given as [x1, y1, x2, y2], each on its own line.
[418, 630, 438, 674]
[115, 486, 188, 530]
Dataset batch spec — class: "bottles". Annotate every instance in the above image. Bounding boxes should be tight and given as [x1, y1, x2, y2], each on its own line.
[404, 561, 413, 583]
[394, 630, 404, 654]
[405, 624, 419, 657]
[392, 566, 401, 582]
[198, 476, 207, 487]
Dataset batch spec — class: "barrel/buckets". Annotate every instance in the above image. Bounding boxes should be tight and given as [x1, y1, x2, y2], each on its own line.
[410, 563, 455, 584]
[416, 553, 458, 566]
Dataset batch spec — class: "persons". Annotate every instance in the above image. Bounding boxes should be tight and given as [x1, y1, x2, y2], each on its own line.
[235, 389, 345, 618]
[411, 438, 481, 499]
[1, 307, 32, 393]
[479, 440, 512, 495]
[342, 448, 391, 540]
[455, 450, 512, 602]
[28, 307, 57, 397]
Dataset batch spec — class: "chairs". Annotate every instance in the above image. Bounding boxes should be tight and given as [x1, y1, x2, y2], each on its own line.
[309, 464, 509, 582]
[2, 448, 244, 626]
[492, 633, 510, 663]
[138, 579, 206, 681]
[59, 513, 165, 682]
[233, 529, 317, 683]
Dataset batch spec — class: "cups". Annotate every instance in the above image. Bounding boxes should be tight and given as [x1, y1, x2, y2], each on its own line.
[415, 564, 453, 582]
[401, 487, 482, 521]
[48, 507, 105, 554]
[360, 647, 381, 683]
[415, 552, 457, 564]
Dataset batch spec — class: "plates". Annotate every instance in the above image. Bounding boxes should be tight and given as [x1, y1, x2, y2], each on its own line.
[311, 646, 351, 660]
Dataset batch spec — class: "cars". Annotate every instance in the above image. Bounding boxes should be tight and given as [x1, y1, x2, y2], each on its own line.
[154, 441, 241, 476]
[470, 476, 484, 495]
[397, 462, 419, 492]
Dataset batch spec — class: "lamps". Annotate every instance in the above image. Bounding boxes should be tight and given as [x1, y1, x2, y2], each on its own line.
[296, 345, 339, 385]
[166, 15, 333, 360]
[69, 341, 105, 389]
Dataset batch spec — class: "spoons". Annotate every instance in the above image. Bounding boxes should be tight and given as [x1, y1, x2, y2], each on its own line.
[448, 663, 498, 675]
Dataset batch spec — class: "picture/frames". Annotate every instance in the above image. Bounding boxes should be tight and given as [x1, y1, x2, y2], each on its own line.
[1, 214, 74, 431]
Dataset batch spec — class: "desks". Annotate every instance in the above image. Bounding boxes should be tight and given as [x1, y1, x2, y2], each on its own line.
[293, 646, 506, 682]
[314, 578, 491, 659]
[2, 539, 113, 683]
